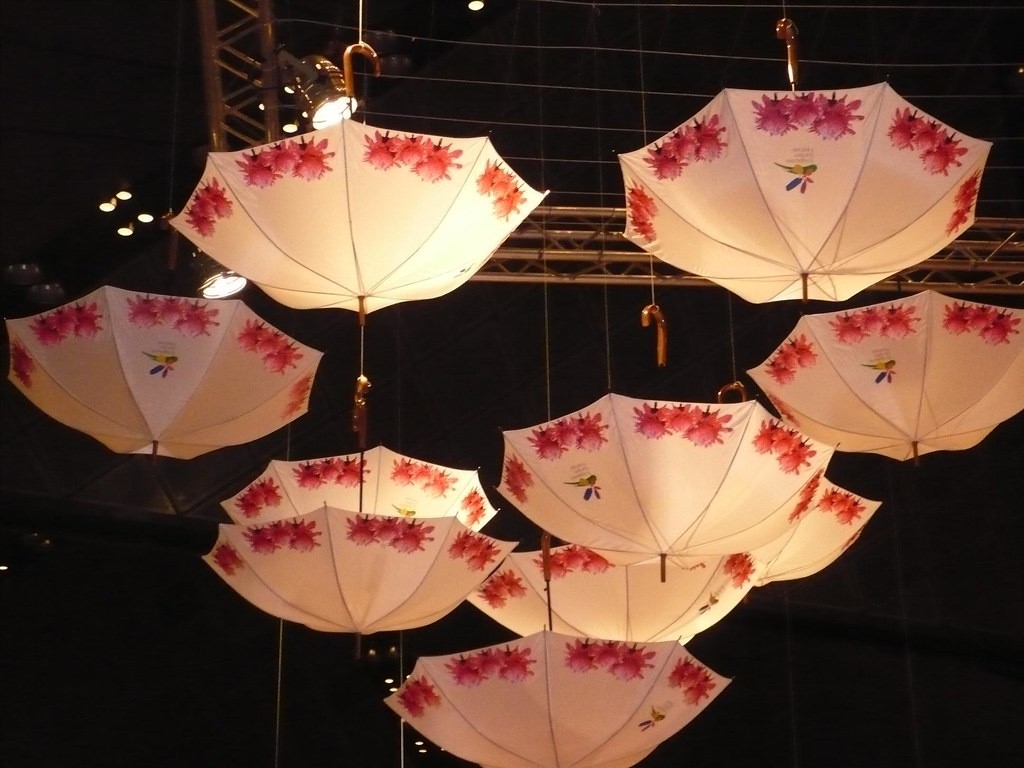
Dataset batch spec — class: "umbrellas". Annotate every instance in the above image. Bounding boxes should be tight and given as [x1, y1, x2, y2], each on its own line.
[746, 288, 1024, 460]
[168, 43, 547, 321]
[203, 304, 883, 768]
[5, 214, 323, 460]
[617, 18, 992, 303]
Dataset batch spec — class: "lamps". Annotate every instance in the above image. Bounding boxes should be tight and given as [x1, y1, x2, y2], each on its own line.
[192, 248, 247, 300]
[278, 51, 358, 130]
